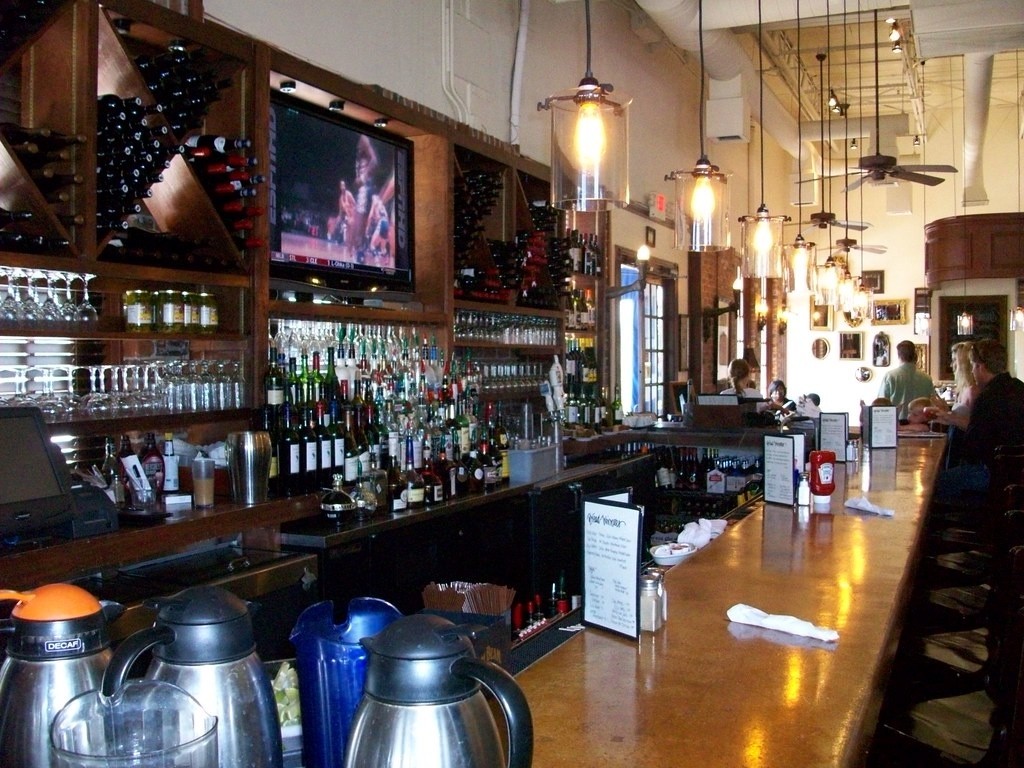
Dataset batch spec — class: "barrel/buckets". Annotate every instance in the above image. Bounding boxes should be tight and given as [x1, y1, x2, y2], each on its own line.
[225, 431, 272, 505]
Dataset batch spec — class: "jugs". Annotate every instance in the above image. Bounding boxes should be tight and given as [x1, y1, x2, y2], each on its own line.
[50, 679, 218, 768]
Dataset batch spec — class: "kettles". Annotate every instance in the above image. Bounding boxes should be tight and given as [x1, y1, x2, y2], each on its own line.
[0, 583, 533, 768]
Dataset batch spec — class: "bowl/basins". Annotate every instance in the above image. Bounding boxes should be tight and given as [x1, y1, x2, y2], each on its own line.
[649, 542, 697, 566]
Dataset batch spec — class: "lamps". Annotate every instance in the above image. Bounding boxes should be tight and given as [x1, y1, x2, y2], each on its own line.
[605, 246, 650, 299]
[704, 278, 742, 343]
[744, 348, 761, 388]
[374, 118, 388, 128]
[779, 311, 789, 335]
[1010, 49, 1024, 332]
[329, 101, 345, 112]
[914, 62, 933, 337]
[544, 0, 634, 212]
[674, 0, 873, 319]
[758, 305, 768, 330]
[958, 59, 973, 336]
[280, 81, 296, 93]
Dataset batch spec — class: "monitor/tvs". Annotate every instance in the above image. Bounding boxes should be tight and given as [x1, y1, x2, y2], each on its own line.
[267, 86, 417, 304]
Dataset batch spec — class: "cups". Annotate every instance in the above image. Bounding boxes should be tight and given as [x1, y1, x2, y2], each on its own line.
[454, 310, 557, 388]
[177, 461, 228, 509]
[127, 480, 158, 509]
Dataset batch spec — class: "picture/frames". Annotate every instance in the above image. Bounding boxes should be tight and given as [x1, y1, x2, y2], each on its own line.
[862, 270, 884, 294]
[838, 331, 864, 362]
[871, 299, 907, 326]
[810, 295, 834, 332]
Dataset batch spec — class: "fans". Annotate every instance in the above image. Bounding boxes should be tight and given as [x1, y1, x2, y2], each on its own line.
[815, 102, 888, 257]
[782, 55, 872, 235]
[795, 10, 958, 193]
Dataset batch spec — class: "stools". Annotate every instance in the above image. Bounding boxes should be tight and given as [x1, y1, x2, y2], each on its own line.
[873, 443, 1024, 768]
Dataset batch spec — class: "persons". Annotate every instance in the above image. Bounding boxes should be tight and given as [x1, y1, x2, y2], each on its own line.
[873, 335, 889, 367]
[927, 338, 1024, 526]
[719, 359, 820, 428]
[860, 340, 936, 434]
[281, 134, 396, 268]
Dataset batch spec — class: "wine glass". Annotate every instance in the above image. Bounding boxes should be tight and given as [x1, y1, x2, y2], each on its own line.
[0, 360, 246, 416]
[0, 267, 99, 332]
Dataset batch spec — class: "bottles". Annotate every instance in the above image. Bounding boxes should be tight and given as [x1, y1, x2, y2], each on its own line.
[564, 337, 624, 430]
[101, 432, 166, 502]
[615, 442, 763, 545]
[566, 281, 573, 330]
[161, 433, 180, 503]
[640, 568, 668, 632]
[573, 288, 595, 330]
[845, 439, 859, 461]
[266, 317, 482, 526]
[571, 229, 601, 277]
[454, 144, 571, 308]
[482, 400, 562, 490]
[514, 574, 582, 642]
[797, 450, 836, 505]
[0, 0, 268, 267]
[121, 290, 221, 335]
